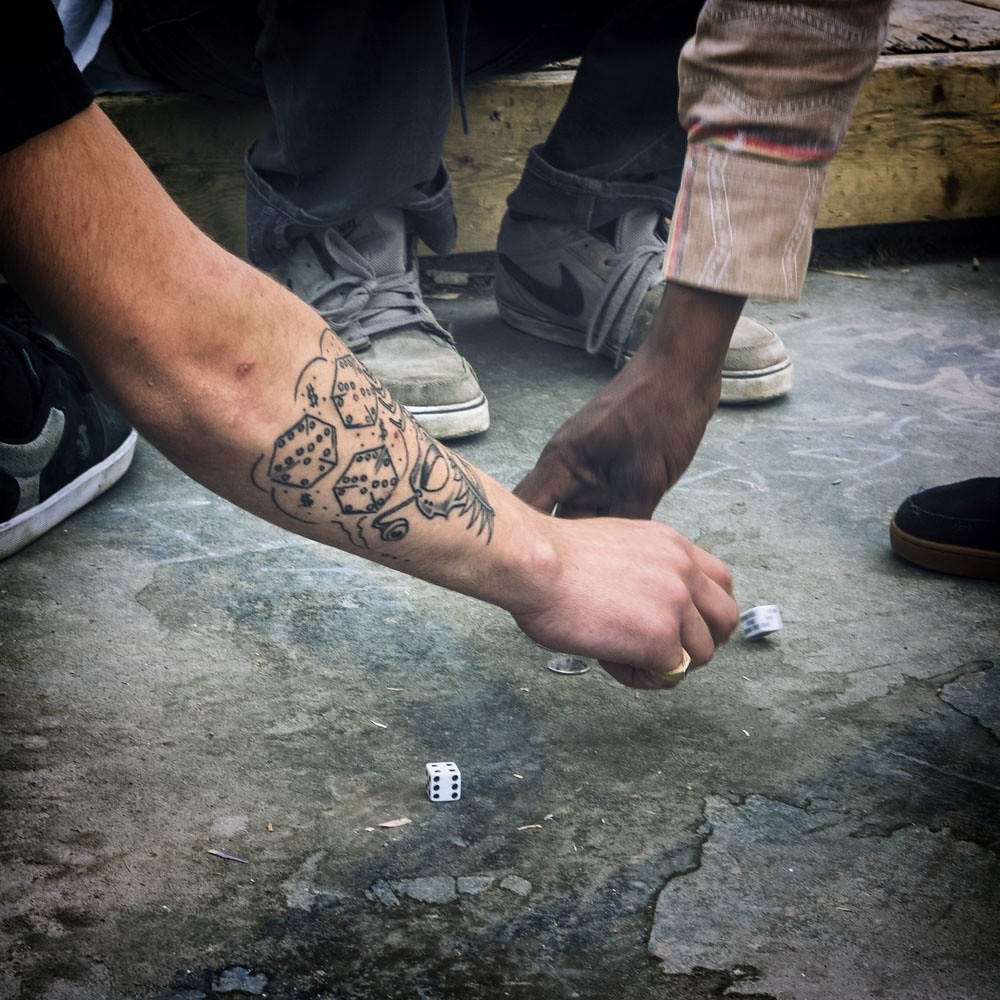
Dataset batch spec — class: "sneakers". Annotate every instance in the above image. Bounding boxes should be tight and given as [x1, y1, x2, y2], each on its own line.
[494, 205, 792, 401]
[0, 325, 138, 558]
[267, 205, 490, 439]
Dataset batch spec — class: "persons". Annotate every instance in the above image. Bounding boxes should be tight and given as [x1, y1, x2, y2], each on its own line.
[0, 0, 739, 695]
[491, 0, 1000, 585]
[245, 0, 797, 437]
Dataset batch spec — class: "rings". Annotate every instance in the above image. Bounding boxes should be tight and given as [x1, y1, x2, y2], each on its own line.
[649, 650, 695, 685]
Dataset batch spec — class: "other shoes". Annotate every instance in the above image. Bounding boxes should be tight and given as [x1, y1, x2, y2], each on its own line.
[888, 474, 1000, 583]
